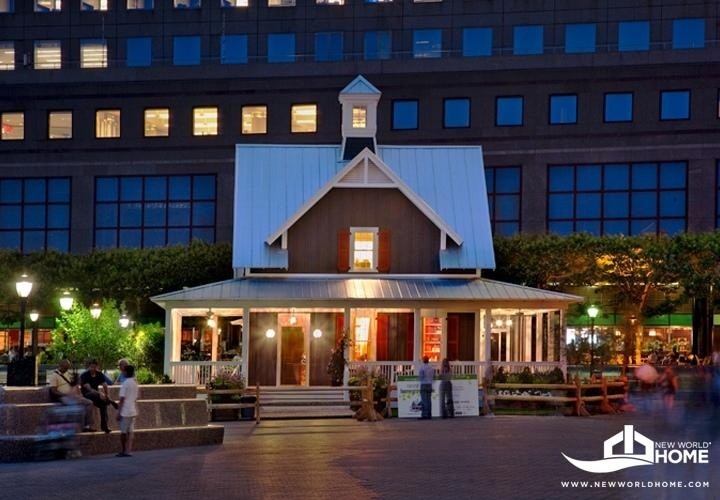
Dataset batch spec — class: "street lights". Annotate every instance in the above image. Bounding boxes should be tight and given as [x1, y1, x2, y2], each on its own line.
[14, 272, 39, 360]
[587, 304, 599, 375]
[58, 291, 72, 358]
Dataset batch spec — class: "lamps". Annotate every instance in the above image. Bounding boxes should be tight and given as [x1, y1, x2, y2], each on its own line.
[288, 308, 296, 325]
[204, 307, 214, 325]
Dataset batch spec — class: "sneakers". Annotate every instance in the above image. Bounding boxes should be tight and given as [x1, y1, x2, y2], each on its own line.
[117, 451, 132, 457]
[100, 425, 112, 432]
[112, 400, 119, 409]
[84, 426, 97, 432]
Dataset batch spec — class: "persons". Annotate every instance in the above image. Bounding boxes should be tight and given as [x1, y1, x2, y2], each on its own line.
[48, 358, 97, 432]
[646, 344, 719, 412]
[418, 355, 435, 419]
[438, 358, 455, 419]
[115, 358, 131, 385]
[8, 345, 49, 365]
[79, 358, 120, 433]
[114, 364, 141, 457]
